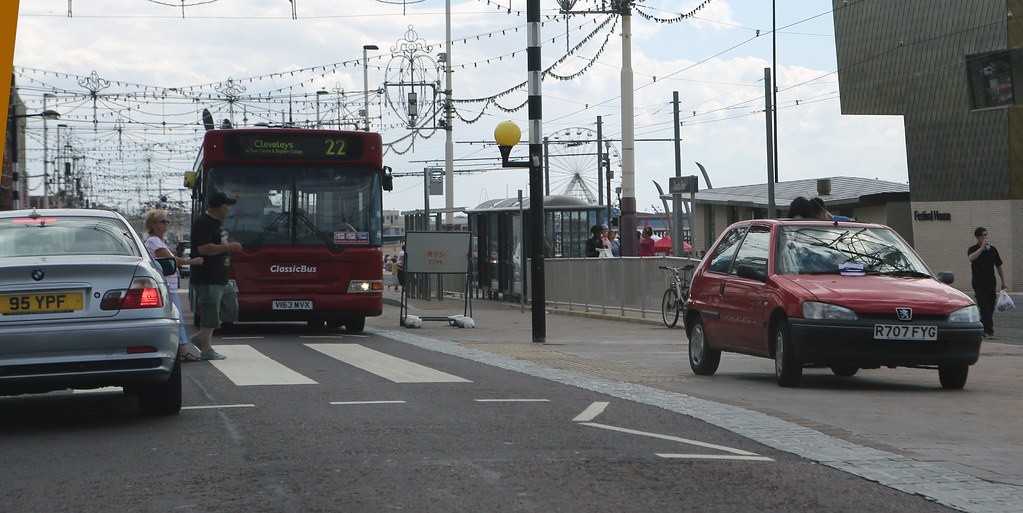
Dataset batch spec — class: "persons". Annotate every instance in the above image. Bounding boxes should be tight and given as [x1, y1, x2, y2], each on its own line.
[809, 197, 850, 221]
[384, 244, 406, 292]
[787, 196, 814, 218]
[190, 193, 242, 360]
[967, 227, 1007, 340]
[144, 209, 203, 362]
[585, 224, 620, 258]
[175, 242, 184, 258]
[636, 226, 655, 256]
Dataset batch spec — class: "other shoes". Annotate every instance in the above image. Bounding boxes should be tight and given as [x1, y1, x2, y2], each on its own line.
[190, 334, 203, 351]
[199, 349, 227, 361]
[179, 351, 200, 362]
[395, 288, 399, 292]
[388, 284, 391, 289]
[985, 331, 995, 338]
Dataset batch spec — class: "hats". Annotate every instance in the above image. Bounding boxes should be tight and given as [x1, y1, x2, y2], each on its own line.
[207, 192, 238, 208]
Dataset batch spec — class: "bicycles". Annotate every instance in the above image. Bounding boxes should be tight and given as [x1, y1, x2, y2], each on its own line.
[659, 264, 695, 328]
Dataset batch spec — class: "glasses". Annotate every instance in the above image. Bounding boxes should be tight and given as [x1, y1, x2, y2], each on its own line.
[152, 218, 171, 225]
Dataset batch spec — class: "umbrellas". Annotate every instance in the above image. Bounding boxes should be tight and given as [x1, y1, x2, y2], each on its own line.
[637, 229, 662, 241]
[654, 236, 691, 256]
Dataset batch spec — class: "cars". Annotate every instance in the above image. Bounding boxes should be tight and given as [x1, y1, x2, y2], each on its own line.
[683, 217, 985, 388]
[179, 248, 191, 279]
[1, 207, 183, 417]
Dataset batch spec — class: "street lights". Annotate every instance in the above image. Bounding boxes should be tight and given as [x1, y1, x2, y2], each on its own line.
[57, 123, 68, 196]
[493, 119, 546, 343]
[316, 90, 329, 130]
[362, 45, 380, 133]
[42, 92, 56, 209]
[64, 144, 73, 192]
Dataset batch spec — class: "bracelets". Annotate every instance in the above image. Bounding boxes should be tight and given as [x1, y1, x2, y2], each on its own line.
[185, 257, 190, 264]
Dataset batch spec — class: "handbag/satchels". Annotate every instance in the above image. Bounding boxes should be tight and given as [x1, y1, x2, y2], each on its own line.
[997, 288, 1015, 313]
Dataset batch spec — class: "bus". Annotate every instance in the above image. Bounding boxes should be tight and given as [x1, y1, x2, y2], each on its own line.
[184, 127, 393, 334]
